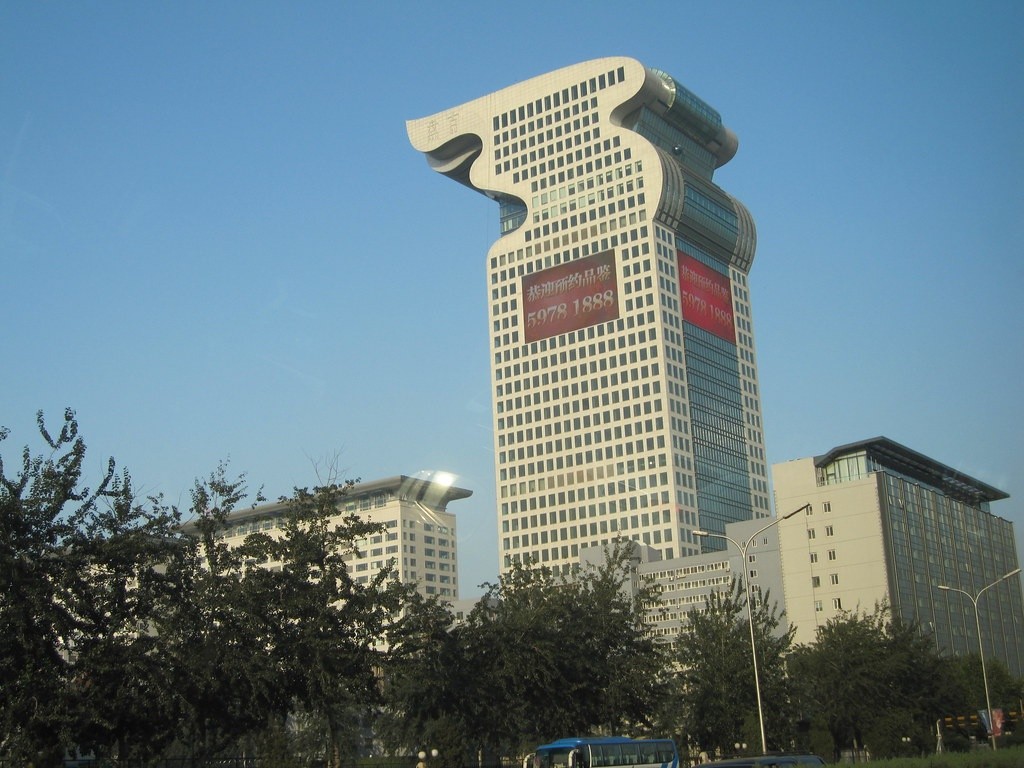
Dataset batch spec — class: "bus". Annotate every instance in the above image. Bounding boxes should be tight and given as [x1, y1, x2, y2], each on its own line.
[523, 735, 679, 768]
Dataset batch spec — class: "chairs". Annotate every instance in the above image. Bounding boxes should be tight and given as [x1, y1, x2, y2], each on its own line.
[593, 753, 674, 766]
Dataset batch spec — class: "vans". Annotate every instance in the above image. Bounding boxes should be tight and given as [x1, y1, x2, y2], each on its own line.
[692, 755, 828, 768]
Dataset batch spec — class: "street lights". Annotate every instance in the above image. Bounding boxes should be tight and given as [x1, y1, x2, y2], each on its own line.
[936, 569, 1022, 752]
[692, 503, 811, 753]
